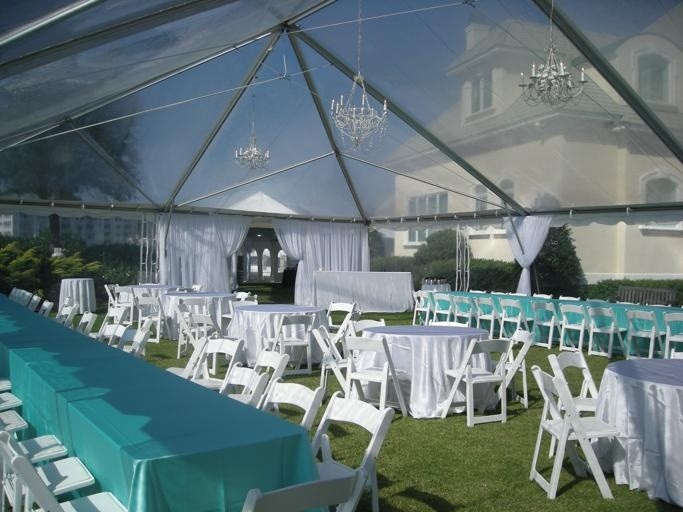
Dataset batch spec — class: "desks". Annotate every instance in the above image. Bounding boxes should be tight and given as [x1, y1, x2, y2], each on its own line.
[594, 357, 683, 508]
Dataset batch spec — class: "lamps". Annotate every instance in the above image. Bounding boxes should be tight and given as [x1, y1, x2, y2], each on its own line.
[232, 80, 272, 169]
[518, 2, 589, 107]
[330, 1, 391, 150]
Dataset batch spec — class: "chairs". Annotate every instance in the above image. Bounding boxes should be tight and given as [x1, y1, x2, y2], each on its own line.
[529, 293, 680, 360]
[546, 349, 604, 458]
[527, 363, 620, 500]
[2, 285, 397, 512]
[101, 282, 535, 424]
